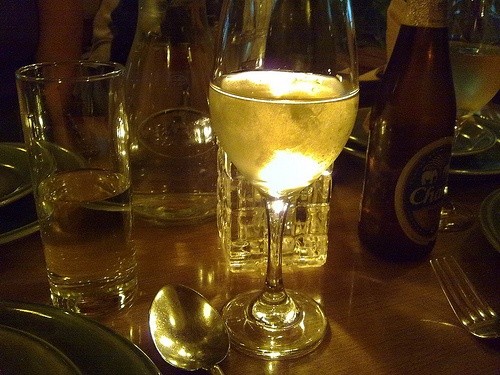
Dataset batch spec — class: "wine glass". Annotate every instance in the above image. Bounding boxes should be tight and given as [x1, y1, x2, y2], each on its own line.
[434, 0, 500, 232]
[207, 0, 360, 361]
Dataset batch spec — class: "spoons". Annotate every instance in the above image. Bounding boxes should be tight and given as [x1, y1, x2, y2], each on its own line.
[149, 282, 231, 375]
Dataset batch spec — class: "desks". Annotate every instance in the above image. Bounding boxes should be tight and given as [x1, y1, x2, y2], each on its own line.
[0, 43, 500, 375]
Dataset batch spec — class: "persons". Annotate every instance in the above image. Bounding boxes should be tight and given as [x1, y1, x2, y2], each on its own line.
[0, 0, 209, 236]
[450, 0, 483, 42]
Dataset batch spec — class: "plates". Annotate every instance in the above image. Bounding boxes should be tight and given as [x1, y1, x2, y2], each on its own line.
[0, 143, 92, 245]
[478, 189, 500, 254]
[341, 106, 500, 174]
[0, 299, 162, 375]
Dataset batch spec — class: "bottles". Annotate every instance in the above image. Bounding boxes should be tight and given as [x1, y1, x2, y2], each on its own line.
[360, 0, 457, 265]
[113, 0, 218, 227]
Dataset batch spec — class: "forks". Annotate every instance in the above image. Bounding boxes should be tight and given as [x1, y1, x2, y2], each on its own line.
[430, 256, 500, 338]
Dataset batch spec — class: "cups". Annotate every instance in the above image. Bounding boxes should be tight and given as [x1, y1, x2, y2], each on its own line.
[217, 144, 332, 274]
[15, 59, 139, 320]
